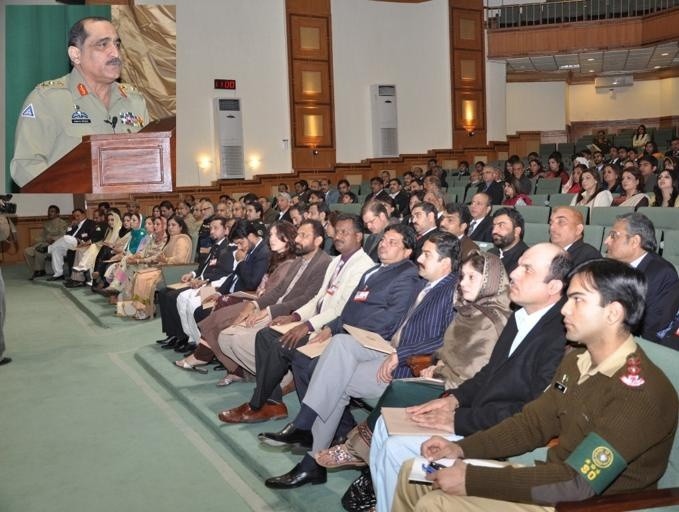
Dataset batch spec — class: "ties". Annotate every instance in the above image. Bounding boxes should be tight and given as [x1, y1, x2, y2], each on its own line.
[391, 283, 433, 349]
[195, 244, 218, 280]
[317, 259, 345, 315]
[364, 265, 387, 290]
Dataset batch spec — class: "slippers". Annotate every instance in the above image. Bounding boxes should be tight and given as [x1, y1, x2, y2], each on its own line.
[172, 358, 209, 375]
[216, 372, 245, 387]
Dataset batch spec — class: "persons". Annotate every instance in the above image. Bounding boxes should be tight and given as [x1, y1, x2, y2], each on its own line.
[0, 199, 17, 366]
[25, 192, 302, 387]
[6, 16, 149, 188]
[218, 122, 679, 512]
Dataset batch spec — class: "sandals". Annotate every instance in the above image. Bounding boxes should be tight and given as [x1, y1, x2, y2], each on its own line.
[91, 281, 158, 321]
[315, 442, 368, 469]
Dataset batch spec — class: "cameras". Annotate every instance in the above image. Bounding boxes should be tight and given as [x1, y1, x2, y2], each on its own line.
[0, 193, 17, 213]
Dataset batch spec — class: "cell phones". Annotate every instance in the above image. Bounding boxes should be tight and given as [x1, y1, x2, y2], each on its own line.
[428, 461, 447, 471]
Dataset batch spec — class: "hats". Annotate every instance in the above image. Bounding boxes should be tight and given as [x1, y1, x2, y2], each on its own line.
[276, 191, 291, 203]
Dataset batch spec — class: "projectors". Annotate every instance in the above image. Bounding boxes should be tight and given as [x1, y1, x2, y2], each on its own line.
[595, 74, 634, 89]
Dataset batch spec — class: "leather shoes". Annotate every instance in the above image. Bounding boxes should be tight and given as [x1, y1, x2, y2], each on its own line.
[30, 269, 46, 281]
[265, 458, 326, 489]
[256, 421, 314, 452]
[63, 279, 86, 288]
[36, 245, 48, 253]
[46, 275, 65, 281]
[218, 400, 255, 424]
[156, 334, 197, 356]
[238, 401, 288, 423]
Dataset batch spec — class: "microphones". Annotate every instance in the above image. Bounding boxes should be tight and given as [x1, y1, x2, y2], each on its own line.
[110, 115, 118, 134]
